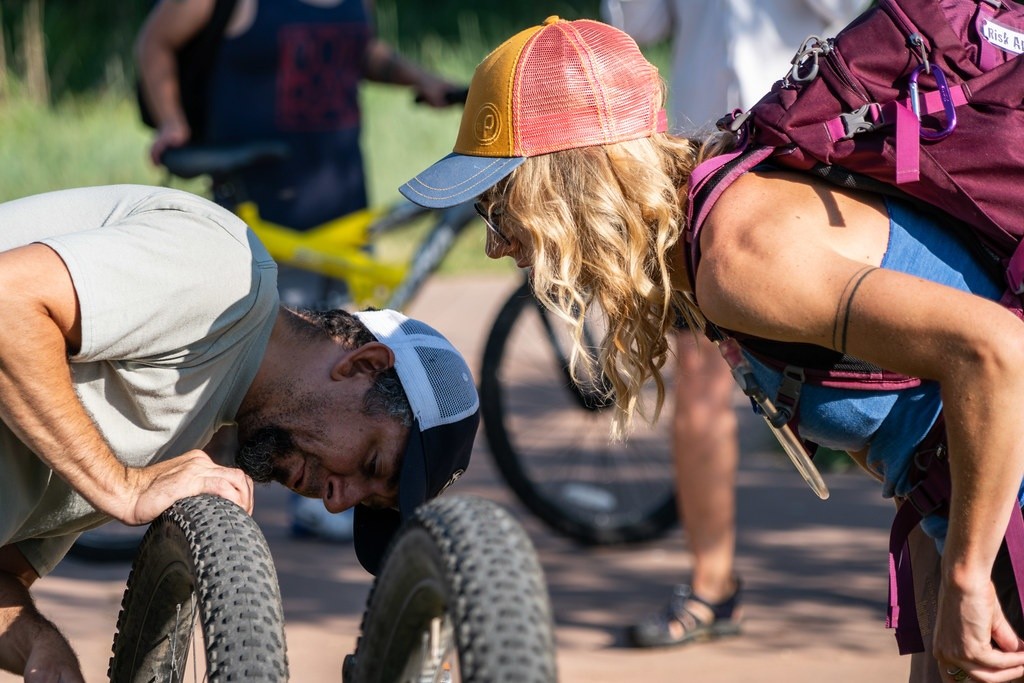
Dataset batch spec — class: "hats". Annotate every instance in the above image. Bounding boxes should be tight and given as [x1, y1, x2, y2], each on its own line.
[346, 308, 480, 577]
[399, 14, 669, 208]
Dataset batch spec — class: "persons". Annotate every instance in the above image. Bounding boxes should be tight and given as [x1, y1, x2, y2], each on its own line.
[114, 0, 459, 538]
[397, 0, 1024, 683]
[0, 181, 481, 683]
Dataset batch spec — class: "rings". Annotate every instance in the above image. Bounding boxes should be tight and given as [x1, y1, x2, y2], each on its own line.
[945, 668, 968, 681]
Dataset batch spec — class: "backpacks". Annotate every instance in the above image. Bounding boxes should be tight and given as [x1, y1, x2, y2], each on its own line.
[682, 0, 1024, 391]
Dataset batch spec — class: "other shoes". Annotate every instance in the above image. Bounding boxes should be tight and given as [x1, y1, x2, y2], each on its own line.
[289, 498, 358, 541]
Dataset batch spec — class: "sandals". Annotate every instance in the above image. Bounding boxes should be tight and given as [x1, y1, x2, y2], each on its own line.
[625, 574, 746, 649]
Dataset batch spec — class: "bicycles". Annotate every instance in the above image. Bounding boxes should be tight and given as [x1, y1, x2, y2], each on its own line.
[63, 78, 685, 566]
